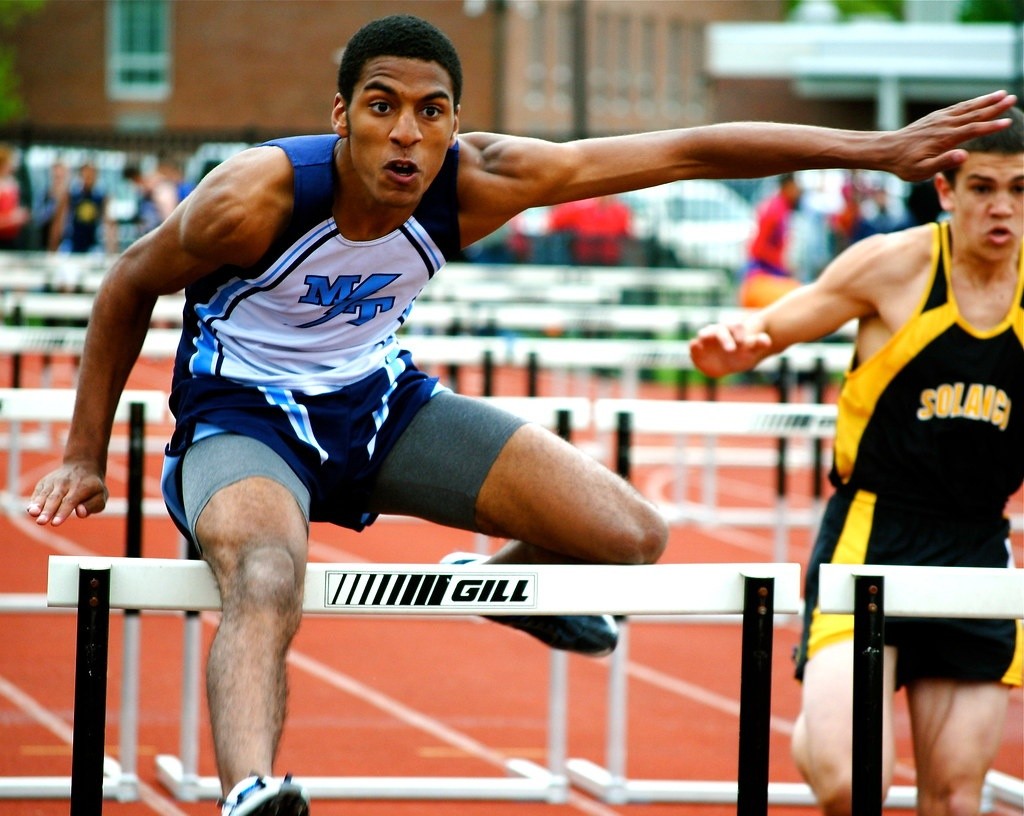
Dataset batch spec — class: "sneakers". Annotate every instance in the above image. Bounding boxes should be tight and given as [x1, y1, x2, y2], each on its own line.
[217, 774, 311, 816]
[437, 551, 616, 658]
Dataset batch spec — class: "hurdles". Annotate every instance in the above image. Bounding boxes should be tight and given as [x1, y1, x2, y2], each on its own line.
[0, 232, 1024, 816]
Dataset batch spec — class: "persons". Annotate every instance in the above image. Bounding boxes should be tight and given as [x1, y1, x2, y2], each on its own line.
[0, 134, 943, 315]
[687, 110, 1024, 816]
[27, 13, 1019, 816]
